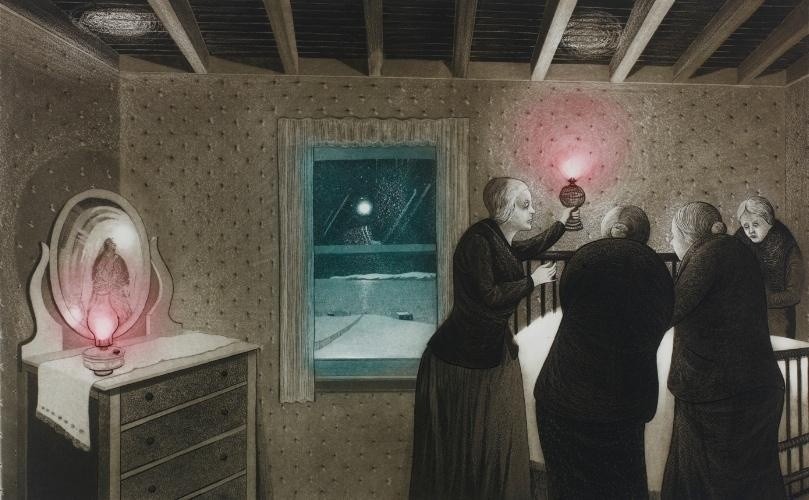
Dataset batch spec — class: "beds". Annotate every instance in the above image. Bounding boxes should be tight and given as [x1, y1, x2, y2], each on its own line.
[514, 254, 807, 500]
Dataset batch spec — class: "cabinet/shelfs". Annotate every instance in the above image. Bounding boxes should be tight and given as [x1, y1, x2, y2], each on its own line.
[19, 354, 257, 500]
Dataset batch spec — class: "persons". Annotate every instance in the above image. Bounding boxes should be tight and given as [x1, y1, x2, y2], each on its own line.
[659, 198, 793, 499]
[410, 178, 579, 500]
[87, 239, 135, 329]
[532, 203, 675, 499]
[733, 197, 805, 341]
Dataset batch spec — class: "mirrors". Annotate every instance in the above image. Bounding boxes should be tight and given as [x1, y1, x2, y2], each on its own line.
[47, 187, 151, 341]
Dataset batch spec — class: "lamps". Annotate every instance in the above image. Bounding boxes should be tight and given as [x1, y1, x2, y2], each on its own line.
[560, 157, 588, 232]
[83, 292, 123, 377]
